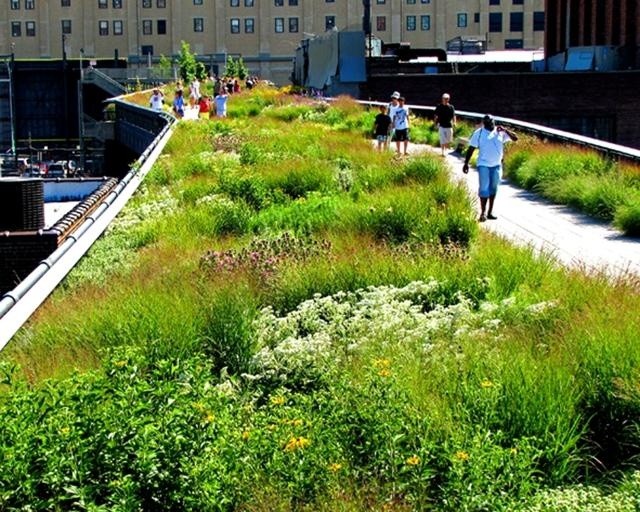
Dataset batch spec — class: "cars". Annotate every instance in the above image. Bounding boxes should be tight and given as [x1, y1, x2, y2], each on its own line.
[0, 157, 76, 178]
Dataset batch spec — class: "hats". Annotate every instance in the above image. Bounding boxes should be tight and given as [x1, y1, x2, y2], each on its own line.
[390, 90, 401, 100]
[442, 92, 450, 100]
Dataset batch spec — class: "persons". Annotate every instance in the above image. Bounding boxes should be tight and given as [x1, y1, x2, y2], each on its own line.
[434, 93, 457, 157]
[387, 91, 401, 150]
[462, 113, 519, 222]
[149, 72, 259, 119]
[393, 97, 409, 154]
[373, 104, 392, 152]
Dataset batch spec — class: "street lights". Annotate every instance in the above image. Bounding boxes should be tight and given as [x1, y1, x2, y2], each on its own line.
[9, 39, 23, 167]
